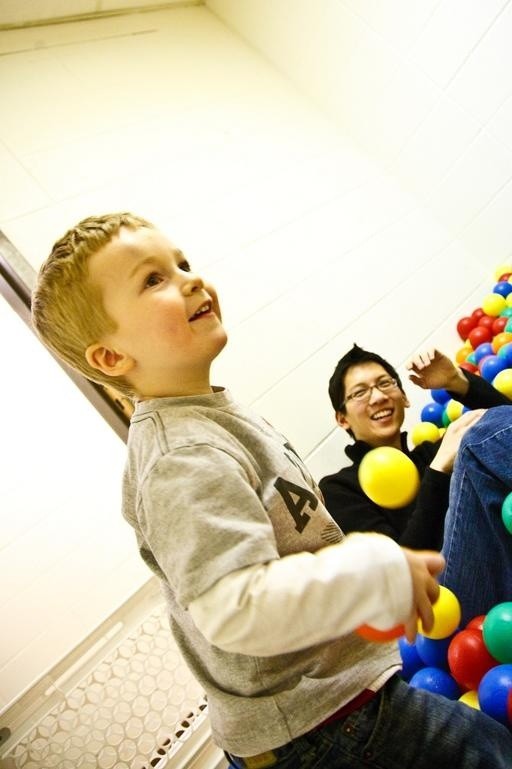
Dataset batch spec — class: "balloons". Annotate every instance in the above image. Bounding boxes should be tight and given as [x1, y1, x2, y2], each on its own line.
[355, 264, 512, 726]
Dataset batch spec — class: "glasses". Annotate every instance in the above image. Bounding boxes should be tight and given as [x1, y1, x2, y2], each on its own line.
[340, 379, 397, 406]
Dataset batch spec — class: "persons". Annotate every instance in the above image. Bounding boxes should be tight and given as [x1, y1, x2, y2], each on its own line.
[28, 211, 512, 767]
[314, 342, 512, 630]
